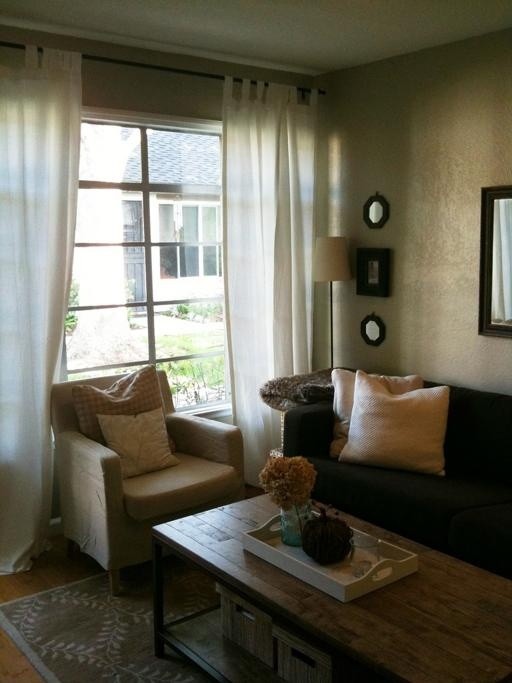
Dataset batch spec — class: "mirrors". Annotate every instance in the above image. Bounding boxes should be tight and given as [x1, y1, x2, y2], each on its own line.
[478, 185, 512, 338]
[363, 195, 389, 228]
[360, 315, 386, 346]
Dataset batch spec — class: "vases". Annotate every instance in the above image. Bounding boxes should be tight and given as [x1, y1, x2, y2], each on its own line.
[280, 502, 313, 547]
[259, 455, 317, 541]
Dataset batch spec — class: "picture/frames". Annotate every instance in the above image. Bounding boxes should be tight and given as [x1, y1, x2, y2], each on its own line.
[356, 249, 390, 297]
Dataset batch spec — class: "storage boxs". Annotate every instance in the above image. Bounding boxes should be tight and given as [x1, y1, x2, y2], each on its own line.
[271, 625, 333, 683]
[215, 582, 276, 669]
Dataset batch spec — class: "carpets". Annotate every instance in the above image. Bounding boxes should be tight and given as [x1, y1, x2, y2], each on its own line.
[0, 555, 221, 683]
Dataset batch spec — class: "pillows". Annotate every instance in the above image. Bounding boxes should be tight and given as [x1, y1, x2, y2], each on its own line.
[330, 369, 450, 476]
[71, 364, 180, 479]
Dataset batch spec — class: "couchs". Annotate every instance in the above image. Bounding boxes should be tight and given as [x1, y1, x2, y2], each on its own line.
[283, 381, 512, 581]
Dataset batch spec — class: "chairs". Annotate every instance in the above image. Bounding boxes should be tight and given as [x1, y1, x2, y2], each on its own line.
[50, 371, 244, 595]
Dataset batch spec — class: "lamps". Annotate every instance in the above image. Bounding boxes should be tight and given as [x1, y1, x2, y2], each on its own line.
[312, 236, 354, 382]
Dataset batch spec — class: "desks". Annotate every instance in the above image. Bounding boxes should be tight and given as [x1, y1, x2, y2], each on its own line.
[151, 493, 512, 683]
